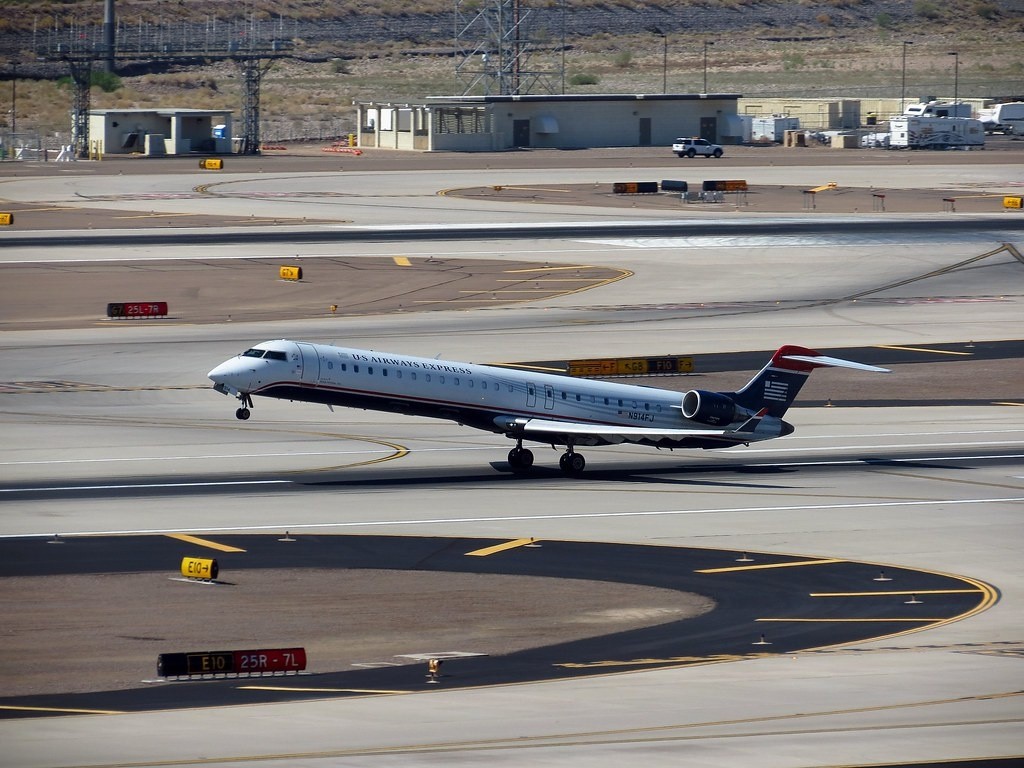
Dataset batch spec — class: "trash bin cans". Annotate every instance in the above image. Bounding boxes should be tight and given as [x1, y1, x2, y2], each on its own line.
[213, 124, 226, 138]
[867, 117, 876, 125]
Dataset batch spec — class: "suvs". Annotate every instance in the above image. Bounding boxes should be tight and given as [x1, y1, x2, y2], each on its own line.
[672, 137, 723, 158]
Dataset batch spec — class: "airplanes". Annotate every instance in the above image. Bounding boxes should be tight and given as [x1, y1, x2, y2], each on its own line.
[207, 338, 893, 474]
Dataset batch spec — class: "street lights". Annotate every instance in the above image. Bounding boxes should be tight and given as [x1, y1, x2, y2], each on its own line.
[948, 53, 958, 117]
[559, 0, 572, 95]
[704, 41, 714, 94]
[661, 35, 666, 94]
[8, 59, 21, 140]
[902, 41, 914, 116]
